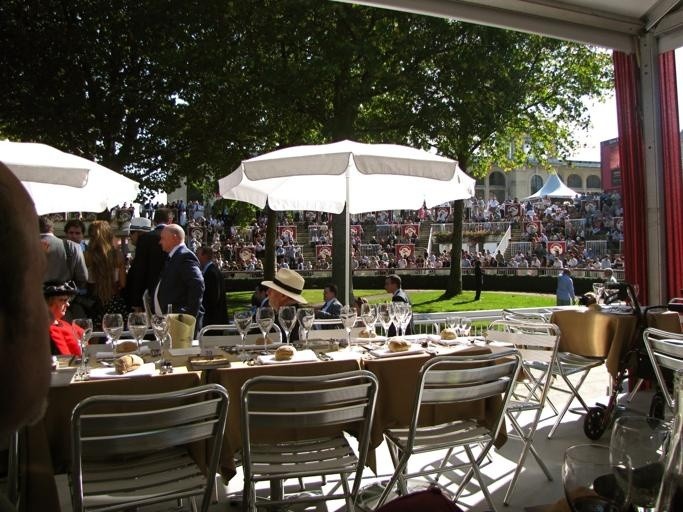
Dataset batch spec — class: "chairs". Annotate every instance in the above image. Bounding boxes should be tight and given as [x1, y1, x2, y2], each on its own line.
[373, 350, 499, 512]
[236, 368, 379, 512]
[642, 325, 683, 413]
[483, 319, 562, 504]
[504, 308, 605, 440]
[53, 383, 231, 512]
[200, 321, 282, 346]
[300, 317, 376, 340]
[76, 329, 173, 355]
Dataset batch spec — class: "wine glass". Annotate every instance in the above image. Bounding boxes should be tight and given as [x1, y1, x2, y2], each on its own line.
[233, 305, 315, 359]
[339, 302, 413, 351]
[446, 316, 472, 335]
[610, 416, 675, 512]
[72, 312, 168, 372]
[562, 442, 632, 512]
[592, 282, 640, 314]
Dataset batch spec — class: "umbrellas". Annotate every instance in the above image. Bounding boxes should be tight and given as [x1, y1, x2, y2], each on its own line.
[218, 139, 476, 310]
[1, 141, 140, 216]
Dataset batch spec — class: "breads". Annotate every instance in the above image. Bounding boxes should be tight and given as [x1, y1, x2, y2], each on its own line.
[275, 345, 297, 360]
[360, 329, 376, 338]
[116, 341, 137, 353]
[441, 328, 457, 340]
[255, 336, 273, 345]
[115, 354, 145, 375]
[389, 339, 411, 353]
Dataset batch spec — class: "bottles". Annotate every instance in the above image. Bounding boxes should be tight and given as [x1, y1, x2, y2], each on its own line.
[178, 314, 183, 323]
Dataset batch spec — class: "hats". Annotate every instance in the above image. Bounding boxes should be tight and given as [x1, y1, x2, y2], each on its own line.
[261, 268, 308, 304]
[123, 218, 152, 232]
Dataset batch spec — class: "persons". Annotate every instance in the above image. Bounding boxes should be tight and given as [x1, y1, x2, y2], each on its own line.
[383, 273, 415, 338]
[149, 224, 207, 341]
[311, 284, 343, 331]
[32, 199, 181, 355]
[250, 268, 307, 344]
[183, 192, 630, 278]
[554, 268, 577, 307]
[602, 268, 617, 286]
[194, 245, 229, 337]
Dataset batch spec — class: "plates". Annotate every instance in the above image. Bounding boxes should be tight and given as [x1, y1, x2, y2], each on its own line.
[87, 363, 155, 378]
[370, 345, 424, 357]
[256, 349, 317, 364]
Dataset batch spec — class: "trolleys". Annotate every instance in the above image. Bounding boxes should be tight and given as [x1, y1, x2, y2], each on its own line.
[581, 282, 679, 442]
